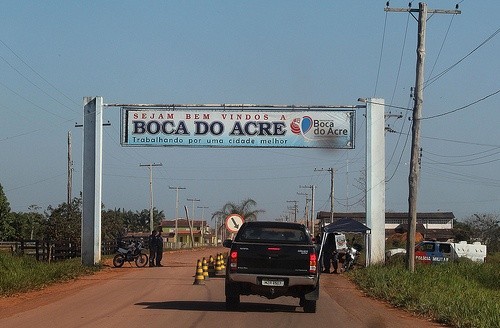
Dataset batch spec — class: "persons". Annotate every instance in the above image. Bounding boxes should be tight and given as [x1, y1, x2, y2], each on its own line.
[321, 232, 338, 274]
[315, 233, 321, 246]
[156, 229, 163, 266]
[148, 229, 158, 267]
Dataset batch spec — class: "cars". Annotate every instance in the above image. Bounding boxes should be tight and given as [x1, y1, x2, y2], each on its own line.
[385, 247, 406, 259]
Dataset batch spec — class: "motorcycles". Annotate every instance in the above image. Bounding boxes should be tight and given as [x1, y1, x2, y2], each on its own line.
[113, 238, 149, 268]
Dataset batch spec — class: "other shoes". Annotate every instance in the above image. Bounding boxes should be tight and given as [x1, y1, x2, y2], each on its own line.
[331, 270, 337, 273]
[157, 264, 162, 266]
[322, 270, 330, 273]
[149, 264, 155, 267]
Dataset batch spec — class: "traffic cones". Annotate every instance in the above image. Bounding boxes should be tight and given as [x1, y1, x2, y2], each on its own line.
[192, 259, 205, 285]
[202, 257, 209, 281]
[214, 253, 226, 271]
[208, 255, 215, 274]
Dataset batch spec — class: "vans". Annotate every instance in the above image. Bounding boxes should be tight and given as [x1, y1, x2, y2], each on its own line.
[415, 241, 454, 263]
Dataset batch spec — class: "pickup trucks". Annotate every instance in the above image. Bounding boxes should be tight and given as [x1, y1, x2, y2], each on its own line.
[223, 221, 320, 313]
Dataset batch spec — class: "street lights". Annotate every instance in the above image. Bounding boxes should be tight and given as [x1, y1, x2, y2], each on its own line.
[357, 97, 423, 274]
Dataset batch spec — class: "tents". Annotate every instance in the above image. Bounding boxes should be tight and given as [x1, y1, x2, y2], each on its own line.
[317, 217, 371, 274]
